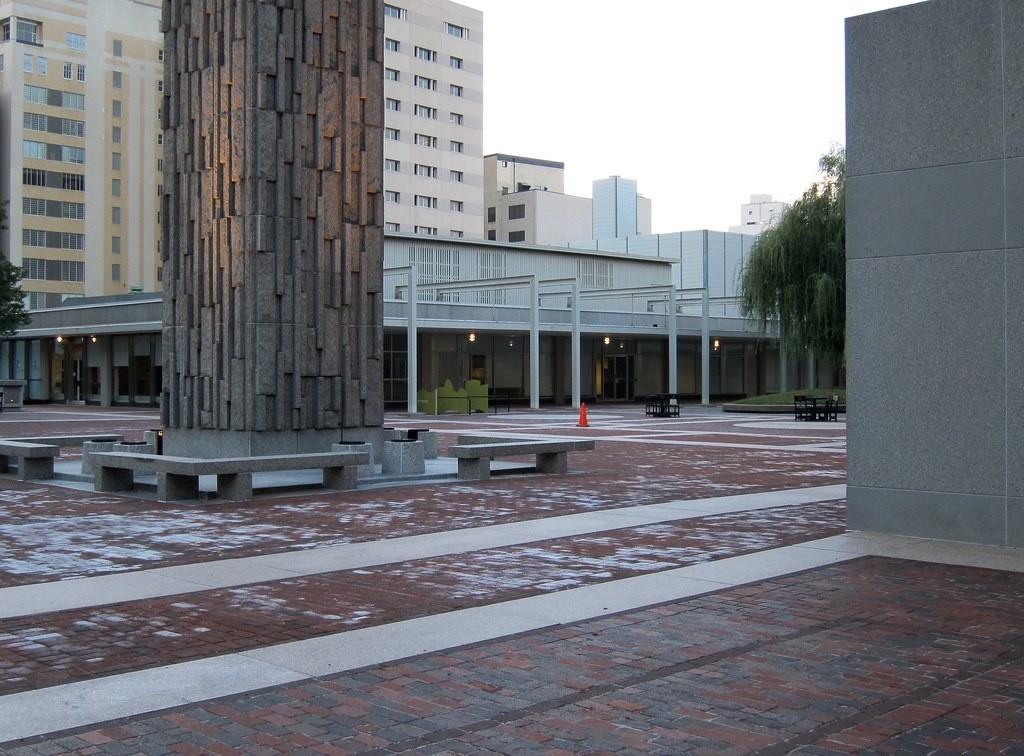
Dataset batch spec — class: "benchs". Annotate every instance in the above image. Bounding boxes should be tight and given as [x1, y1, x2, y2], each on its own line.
[0, 430, 369, 502]
[449, 434, 594, 480]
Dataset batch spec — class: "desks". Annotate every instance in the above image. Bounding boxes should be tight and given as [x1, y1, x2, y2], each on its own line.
[807, 397, 828, 422]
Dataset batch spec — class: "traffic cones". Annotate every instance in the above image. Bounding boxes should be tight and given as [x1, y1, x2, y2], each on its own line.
[576, 402, 590, 428]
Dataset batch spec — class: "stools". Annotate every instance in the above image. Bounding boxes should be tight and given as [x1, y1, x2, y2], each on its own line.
[112, 444, 155, 475]
[330, 443, 375, 479]
[382, 440, 425, 475]
[81, 441, 117, 477]
[383, 430, 400, 441]
[406, 431, 438, 459]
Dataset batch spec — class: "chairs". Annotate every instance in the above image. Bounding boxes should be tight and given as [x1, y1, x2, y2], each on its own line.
[794, 394, 839, 422]
[645, 393, 681, 418]
